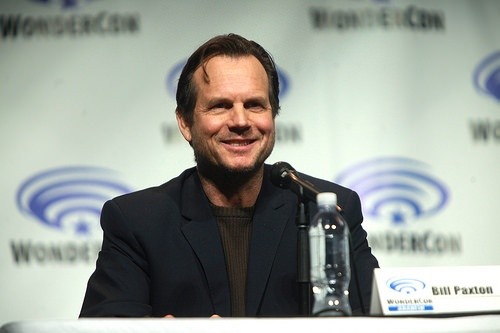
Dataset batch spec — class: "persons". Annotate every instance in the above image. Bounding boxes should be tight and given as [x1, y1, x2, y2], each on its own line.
[77, 32, 380, 318]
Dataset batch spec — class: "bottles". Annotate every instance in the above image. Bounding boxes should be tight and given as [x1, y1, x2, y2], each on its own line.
[310, 192, 353, 316]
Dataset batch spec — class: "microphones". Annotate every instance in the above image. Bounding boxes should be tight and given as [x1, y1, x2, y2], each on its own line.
[270, 162, 342, 213]
